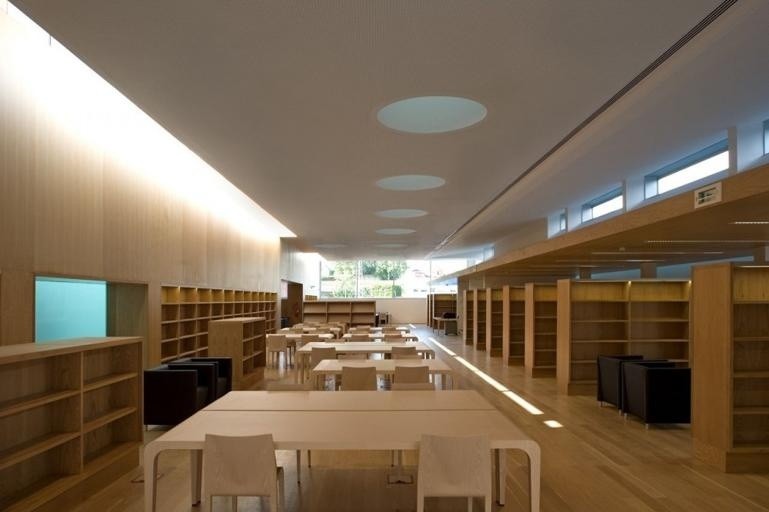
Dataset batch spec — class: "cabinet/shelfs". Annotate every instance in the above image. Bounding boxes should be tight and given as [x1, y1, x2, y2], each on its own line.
[473, 288, 486, 352]
[597, 353, 667, 414]
[0, 335, 144, 512]
[556, 279, 631, 397]
[630, 281, 691, 367]
[427, 293, 458, 335]
[463, 290, 473, 345]
[486, 287, 503, 358]
[525, 282, 556, 379]
[159, 284, 278, 390]
[208, 318, 266, 376]
[302, 300, 377, 328]
[691, 261, 768, 474]
[502, 285, 525, 368]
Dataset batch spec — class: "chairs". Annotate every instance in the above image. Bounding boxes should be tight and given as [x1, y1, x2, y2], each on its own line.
[311, 347, 337, 373]
[416, 433, 494, 512]
[623, 360, 692, 428]
[201, 432, 285, 511]
[268, 320, 418, 368]
[391, 365, 435, 391]
[390, 346, 422, 359]
[341, 366, 377, 390]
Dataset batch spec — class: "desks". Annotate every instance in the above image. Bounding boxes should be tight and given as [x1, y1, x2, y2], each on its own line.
[293, 341, 436, 384]
[144, 357, 233, 432]
[143, 410, 542, 511]
[312, 359, 454, 391]
